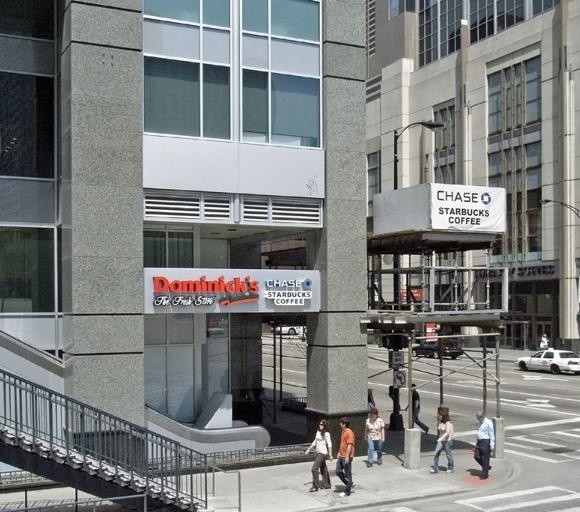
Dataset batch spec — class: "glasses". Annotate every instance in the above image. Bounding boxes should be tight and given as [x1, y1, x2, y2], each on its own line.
[318, 424, 323, 426]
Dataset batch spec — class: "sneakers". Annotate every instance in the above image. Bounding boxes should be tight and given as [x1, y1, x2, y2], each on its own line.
[480, 464, 492, 480]
[309, 485, 332, 492]
[368, 460, 382, 467]
[339, 481, 357, 498]
[430, 469, 453, 473]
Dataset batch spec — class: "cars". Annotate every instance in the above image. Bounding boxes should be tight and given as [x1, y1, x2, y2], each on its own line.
[412, 340, 463, 360]
[271, 323, 308, 335]
[514, 348, 580, 376]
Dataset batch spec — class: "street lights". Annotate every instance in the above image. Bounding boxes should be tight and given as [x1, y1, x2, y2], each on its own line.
[387, 120, 445, 432]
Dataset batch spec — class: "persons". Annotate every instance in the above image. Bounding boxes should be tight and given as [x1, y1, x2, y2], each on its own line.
[473, 410, 495, 480]
[428, 409, 454, 474]
[335, 417, 357, 497]
[405, 383, 430, 437]
[304, 419, 334, 493]
[365, 407, 386, 468]
[368, 396, 374, 411]
[541, 333, 549, 349]
